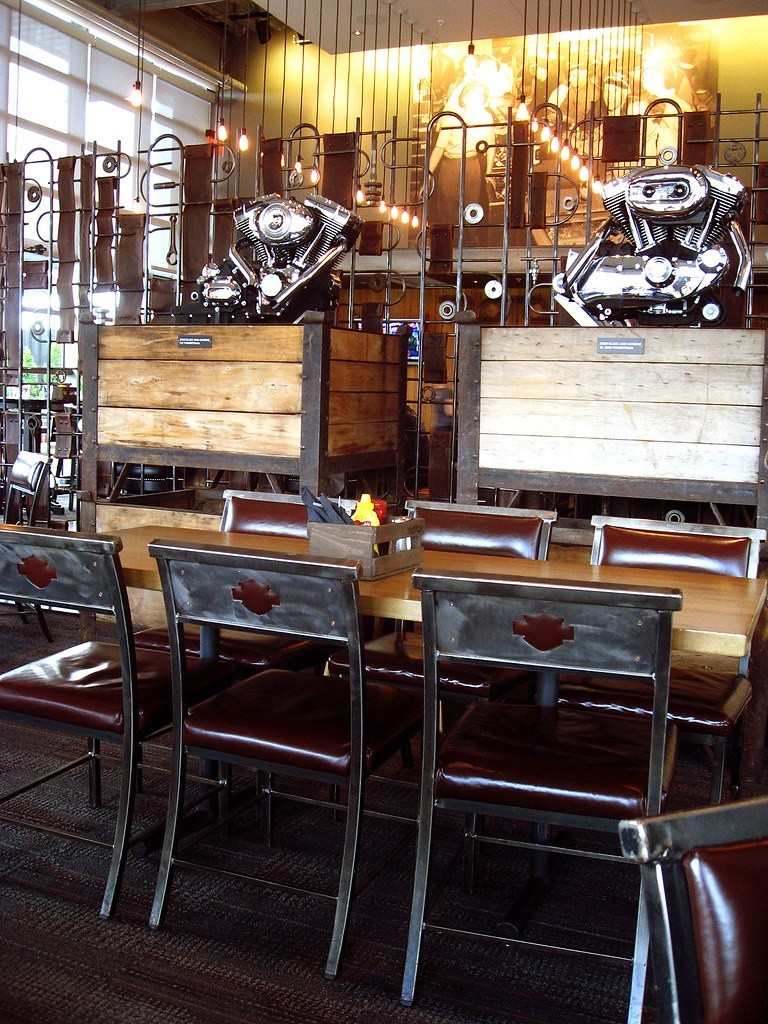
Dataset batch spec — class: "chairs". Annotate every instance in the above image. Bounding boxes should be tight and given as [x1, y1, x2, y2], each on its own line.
[1, 451, 768, 1024]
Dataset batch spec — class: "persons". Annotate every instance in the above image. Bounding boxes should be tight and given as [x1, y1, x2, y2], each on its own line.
[331, 405, 429, 501]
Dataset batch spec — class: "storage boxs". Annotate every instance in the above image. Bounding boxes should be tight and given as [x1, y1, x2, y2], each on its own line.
[307, 518, 425, 582]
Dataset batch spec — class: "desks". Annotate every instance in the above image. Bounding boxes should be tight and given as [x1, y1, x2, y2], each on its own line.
[85, 525, 768, 802]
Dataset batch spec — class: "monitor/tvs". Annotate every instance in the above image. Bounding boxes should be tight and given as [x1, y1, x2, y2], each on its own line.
[354, 319, 424, 365]
